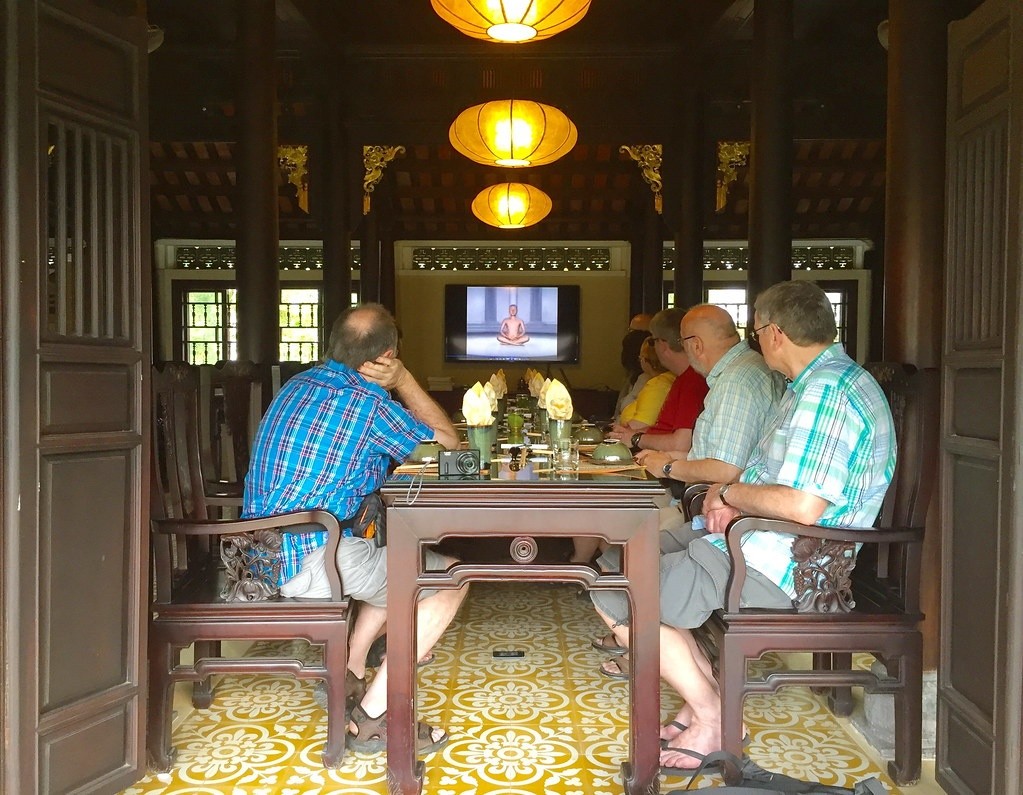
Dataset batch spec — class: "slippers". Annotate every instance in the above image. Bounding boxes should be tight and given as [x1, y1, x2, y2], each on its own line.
[660, 720, 750, 777]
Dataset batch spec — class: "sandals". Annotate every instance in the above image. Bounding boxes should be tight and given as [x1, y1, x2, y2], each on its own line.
[591, 632, 628, 654]
[346, 706, 449, 755]
[368, 634, 435, 668]
[313, 667, 368, 724]
[600, 655, 632, 677]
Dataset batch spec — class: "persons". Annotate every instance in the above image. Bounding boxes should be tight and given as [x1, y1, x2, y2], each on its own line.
[539, 304, 786, 674]
[240, 301, 470, 755]
[591, 281, 900, 777]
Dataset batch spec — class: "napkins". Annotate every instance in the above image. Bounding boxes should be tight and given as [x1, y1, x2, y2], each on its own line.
[522, 368, 572, 421]
[461, 367, 508, 428]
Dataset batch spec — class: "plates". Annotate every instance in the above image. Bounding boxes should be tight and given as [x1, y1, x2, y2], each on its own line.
[570, 444, 597, 452]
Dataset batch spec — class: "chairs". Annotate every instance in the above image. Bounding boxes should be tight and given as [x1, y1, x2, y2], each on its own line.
[674, 354, 945, 787]
[145, 362, 376, 769]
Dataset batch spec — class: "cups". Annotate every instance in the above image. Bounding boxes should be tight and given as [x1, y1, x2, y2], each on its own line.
[552, 438, 579, 472]
[467, 392, 571, 466]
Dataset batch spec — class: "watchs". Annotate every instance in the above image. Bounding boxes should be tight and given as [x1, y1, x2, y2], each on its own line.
[719, 483, 732, 504]
[662, 458, 679, 479]
[631, 432, 647, 450]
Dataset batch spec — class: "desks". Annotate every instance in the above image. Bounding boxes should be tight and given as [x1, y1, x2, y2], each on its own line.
[376, 404, 668, 795]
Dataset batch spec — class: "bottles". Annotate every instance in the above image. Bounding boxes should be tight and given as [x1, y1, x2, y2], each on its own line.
[517, 378, 526, 391]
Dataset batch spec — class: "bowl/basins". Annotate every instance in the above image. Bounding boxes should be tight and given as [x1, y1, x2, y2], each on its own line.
[592, 438, 632, 461]
[573, 426, 603, 445]
[571, 409, 579, 423]
[452, 408, 466, 423]
[410, 440, 447, 462]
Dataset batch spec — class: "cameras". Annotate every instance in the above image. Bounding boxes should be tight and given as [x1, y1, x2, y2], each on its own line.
[438, 449, 480, 476]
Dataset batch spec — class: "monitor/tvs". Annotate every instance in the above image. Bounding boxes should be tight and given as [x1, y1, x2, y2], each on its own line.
[445, 284, 581, 364]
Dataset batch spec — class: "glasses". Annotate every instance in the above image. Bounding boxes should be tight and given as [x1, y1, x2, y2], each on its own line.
[647, 337, 668, 347]
[748, 323, 783, 341]
[676, 335, 695, 345]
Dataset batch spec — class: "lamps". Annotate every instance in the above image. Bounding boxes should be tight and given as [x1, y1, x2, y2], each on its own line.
[471, 183, 553, 228]
[430, 0, 591, 44]
[448, 99, 578, 168]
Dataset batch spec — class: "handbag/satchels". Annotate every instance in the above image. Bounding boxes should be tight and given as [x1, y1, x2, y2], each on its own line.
[665, 751, 888, 795]
[352, 493, 385, 539]
